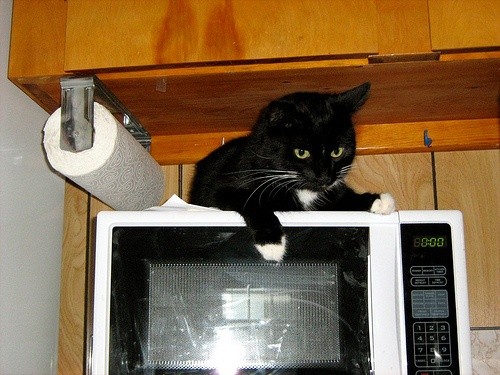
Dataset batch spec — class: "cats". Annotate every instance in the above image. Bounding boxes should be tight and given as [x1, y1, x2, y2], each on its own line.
[186, 80, 397, 263]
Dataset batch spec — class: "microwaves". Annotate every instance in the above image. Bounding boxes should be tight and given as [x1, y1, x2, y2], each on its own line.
[90, 209, 473, 375]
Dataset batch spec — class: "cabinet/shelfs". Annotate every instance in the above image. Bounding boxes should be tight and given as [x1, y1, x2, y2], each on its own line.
[57, 135, 181, 375]
[6, 1, 499, 167]
[434, 117, 500, 329]
[182, 120, 435, 228]
